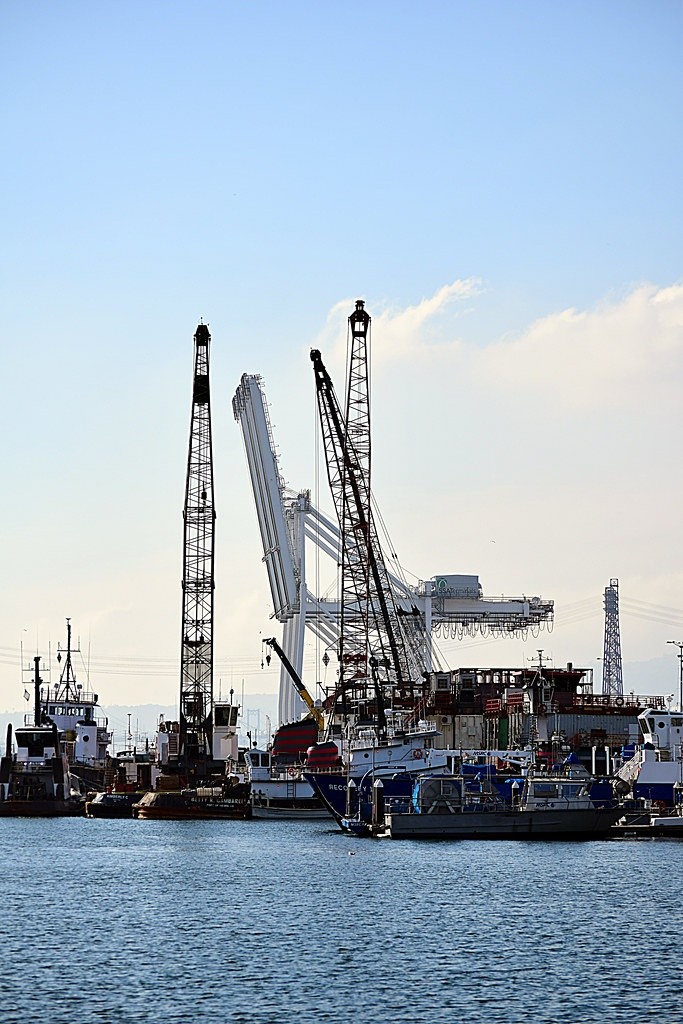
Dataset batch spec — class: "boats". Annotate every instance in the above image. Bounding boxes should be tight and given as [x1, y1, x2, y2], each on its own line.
[0, 617, 114, 816]
[610, 707, 683, 806]
[130, 790, 254, 818]
[85, 786, 145, 819]
[298, 650, 632, 837]
[244, 657, 451, 803]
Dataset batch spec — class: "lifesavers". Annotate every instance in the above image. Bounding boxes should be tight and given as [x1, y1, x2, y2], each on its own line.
[288, 767, 297, 776]
[653, 800, 666, 813]
[413, 749, 423, 759]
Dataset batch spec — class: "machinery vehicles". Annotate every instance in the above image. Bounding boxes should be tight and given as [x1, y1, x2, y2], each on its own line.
[262, 637, 353, 735]
